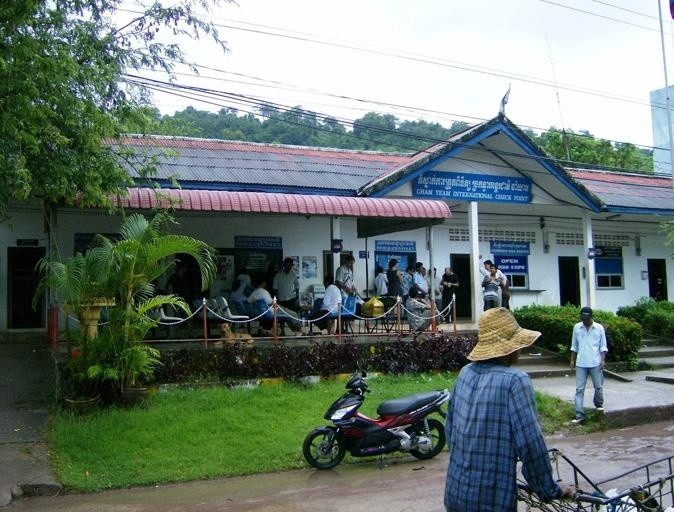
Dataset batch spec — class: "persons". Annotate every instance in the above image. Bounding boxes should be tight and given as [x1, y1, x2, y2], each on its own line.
[374, 257, 460, 330]
[568, 307, 609, 423]
[155, 253, 359, 336]
[480, 259, 512, 312]
[442, 306, 576, 512]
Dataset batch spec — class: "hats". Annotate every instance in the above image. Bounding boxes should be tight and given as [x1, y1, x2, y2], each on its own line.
[284, 257, 295, 267]
[466, 305, 543, 364]
[579, 307, 592, 321]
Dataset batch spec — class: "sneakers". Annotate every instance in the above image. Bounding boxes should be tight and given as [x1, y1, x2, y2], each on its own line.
[595, 407, 604, 414]
[571, 417, 585, 424]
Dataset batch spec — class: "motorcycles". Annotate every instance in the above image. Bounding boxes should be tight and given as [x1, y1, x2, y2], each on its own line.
[302, 370, 453, 471]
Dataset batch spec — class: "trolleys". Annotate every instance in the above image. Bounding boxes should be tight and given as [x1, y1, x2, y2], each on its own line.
[517, 446, 674, 512]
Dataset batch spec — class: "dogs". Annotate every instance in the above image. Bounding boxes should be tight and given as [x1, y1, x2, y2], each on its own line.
[213, 322, 254, 346]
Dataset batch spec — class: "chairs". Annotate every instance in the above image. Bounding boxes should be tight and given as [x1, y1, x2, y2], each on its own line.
[154, 295, 440, 337]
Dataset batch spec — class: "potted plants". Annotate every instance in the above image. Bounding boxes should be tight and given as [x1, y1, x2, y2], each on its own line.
[34, 243, 105, 416]
[89, 203, 219, 410]
